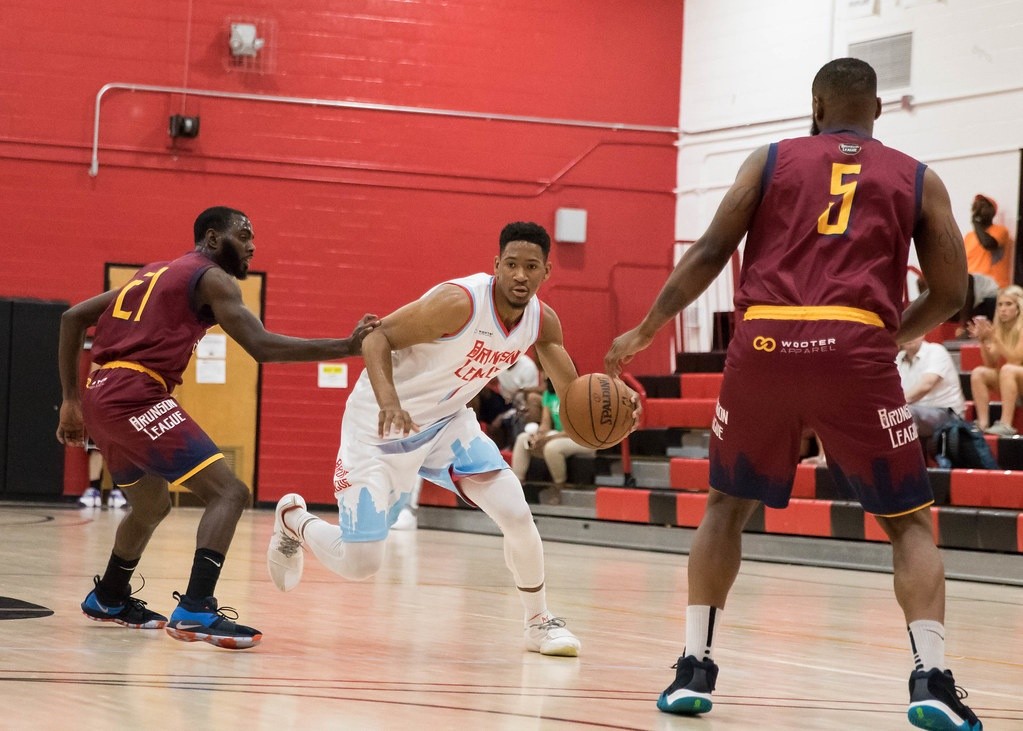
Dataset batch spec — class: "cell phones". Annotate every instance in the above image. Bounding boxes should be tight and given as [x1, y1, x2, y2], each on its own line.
[524, 440, 532, 450]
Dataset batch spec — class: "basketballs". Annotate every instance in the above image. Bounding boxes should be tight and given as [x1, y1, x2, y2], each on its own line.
[559, 372, 637, 449]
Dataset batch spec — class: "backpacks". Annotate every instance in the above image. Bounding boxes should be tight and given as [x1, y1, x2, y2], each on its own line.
[925, 407, 1000, 469]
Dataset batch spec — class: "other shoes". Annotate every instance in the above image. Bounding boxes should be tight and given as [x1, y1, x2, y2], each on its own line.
[79, 486, 102, 508]
[547, 481, 566, 494]
[984, 422, 1018, 435]
[954, 328, 970, 340]
[107, 490, 129, 509]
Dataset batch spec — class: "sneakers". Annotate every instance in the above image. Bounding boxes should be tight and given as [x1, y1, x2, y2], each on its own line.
[267, 493, 307, 593]
[166, 591, 262, 650]
[907, 666, 983, 731]
[656, 647, 719, 713]
[81, 575, 168, 629]
[524, 612, 580, 657]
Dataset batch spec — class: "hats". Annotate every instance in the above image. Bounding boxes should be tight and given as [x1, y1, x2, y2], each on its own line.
[975, 194, 998, 218]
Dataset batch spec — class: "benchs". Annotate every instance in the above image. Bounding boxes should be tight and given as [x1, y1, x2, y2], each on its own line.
[416, 300, 1023, 552]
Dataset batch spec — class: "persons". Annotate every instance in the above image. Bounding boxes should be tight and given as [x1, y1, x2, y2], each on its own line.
[917, 271, 998, 332]
[968, 285, 1023, 435]
[266, 222, 641, 658]
[468, 358, 598, 492]
[962, 193, 1011, 291]
[54, 206, 380, 649]
[893, 331, 966, 439]
[605, 57, 984, 731]
[77, 434, 130, 508]
